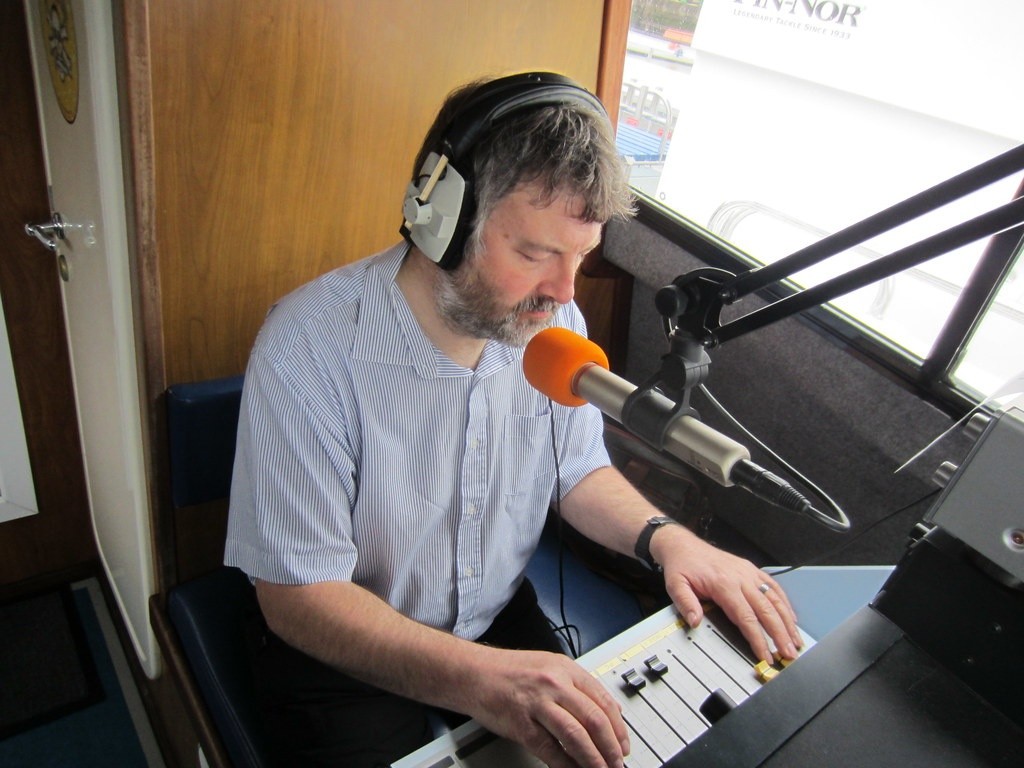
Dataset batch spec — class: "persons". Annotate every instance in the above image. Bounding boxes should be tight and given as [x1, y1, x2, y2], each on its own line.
[223, 71, 804, 768]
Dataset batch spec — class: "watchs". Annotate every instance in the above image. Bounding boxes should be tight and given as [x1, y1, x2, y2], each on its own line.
[634, 518, 679, 574]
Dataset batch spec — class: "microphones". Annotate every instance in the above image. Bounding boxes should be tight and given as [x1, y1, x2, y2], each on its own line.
[522, 325, 812, 520]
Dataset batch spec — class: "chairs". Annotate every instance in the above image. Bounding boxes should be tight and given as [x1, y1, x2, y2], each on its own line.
[148, 380, 717, 768]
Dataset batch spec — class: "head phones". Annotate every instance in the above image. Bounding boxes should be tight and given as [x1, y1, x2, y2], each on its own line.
[398, 72, 620, 273]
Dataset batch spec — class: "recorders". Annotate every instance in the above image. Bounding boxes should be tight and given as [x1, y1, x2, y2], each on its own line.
[864, 409, 1024, 739]
